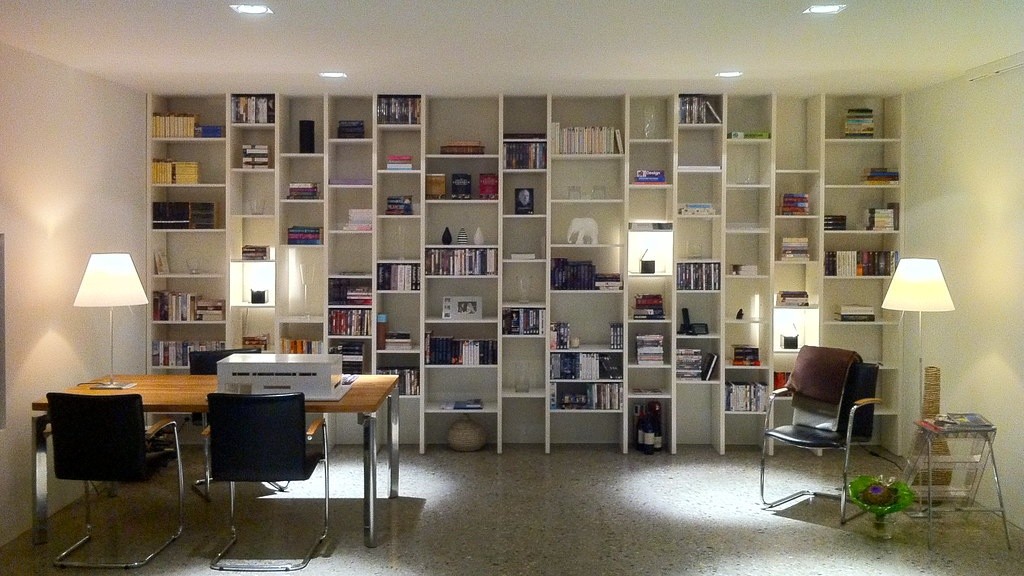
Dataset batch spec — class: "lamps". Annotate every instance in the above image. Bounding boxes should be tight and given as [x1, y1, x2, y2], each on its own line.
[74, 253, 149, 389]
[881, 259, 955, 506]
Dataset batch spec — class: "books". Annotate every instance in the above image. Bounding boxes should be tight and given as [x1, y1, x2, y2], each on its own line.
[680, 97, 771, 139]
[152, 338, 225, 366]
[152, 158, 201, 183]
[152, 113, 224, 139]
[549, 259, 626, 411]
[780, 192, 810, 306]
[242, 245, 269, 261]
[681, 259, 760, 290]
[632, 295, 769, 453]
[378, 96, 498, 215]
[822, 106, 900, 323]
[284, 182, 324, 246]
[242, 336, 322, 355]
[506, 122, 622, 170]
[152, 200, 216, 229]
[91, 382, 137, 390]
[326, 249, 625, 412]
[923, 409, 996, 434]
[630, 170, 714, 230]
[232, 95, 275, 169]
[515, 188, 534, 213]
[154, 289, 225, 322]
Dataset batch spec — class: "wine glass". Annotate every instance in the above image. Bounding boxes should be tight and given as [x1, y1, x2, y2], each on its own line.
[300, 262, 316, 321]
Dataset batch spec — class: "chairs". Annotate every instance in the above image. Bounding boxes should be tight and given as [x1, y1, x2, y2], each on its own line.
[189, 348, 261, 503]
[760, 345, 878, 524]
[46, 391, 184, 567]
[208, 392, 330, 572]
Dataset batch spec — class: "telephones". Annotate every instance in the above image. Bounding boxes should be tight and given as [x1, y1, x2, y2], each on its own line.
[680, 307, 709, 335]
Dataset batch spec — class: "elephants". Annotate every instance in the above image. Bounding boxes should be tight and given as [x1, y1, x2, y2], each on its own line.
[566, 217, 599, 245]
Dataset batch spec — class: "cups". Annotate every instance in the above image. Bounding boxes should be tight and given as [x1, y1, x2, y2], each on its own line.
[590, 185, 606, 200]
[636, 406, 662, 456]
[249, 198, 267, 216]
[568, 186, 582, 199]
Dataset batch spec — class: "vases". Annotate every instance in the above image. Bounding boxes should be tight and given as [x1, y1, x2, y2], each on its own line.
[917, 366, 953, 504]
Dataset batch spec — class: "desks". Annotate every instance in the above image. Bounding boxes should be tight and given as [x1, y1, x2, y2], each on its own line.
[33, 375, 400, 548]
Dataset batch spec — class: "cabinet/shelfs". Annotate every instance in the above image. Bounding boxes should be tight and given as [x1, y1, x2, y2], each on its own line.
[897, 413, 1015, 552]
[145, 93, 906, 454]
